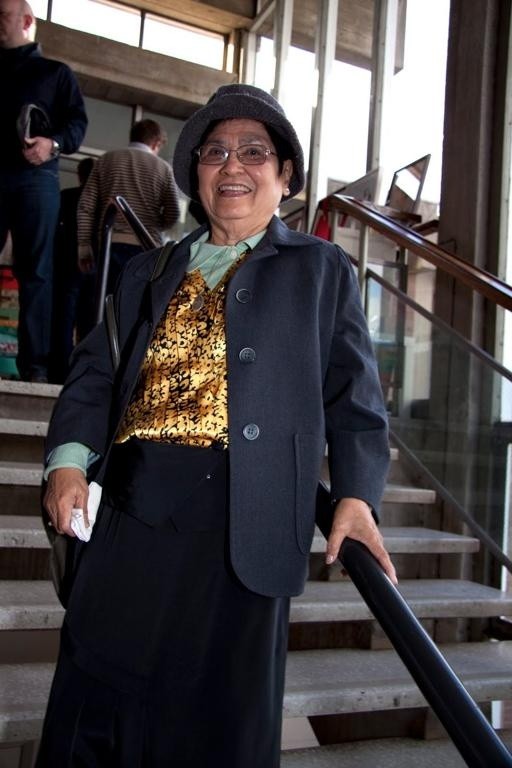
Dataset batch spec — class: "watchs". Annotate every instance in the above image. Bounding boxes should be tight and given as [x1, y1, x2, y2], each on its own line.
[50, 139, 60, 157]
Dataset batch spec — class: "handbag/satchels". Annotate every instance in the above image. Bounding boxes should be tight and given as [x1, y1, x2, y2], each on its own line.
[39, 469, 95, 611]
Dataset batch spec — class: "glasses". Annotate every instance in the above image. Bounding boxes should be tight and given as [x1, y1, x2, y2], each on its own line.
[195, 143, 278, 166]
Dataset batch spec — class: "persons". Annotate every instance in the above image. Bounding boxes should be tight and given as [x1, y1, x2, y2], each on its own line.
[52, 157, 98, 384]
[78, 117, 182, 342]
[31, 85, 399, 768]
[1, 1, 90, 384]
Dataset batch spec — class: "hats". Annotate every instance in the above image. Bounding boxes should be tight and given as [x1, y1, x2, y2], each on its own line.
[16, 103, 52, 150]
[173, 82, 306, 206]
